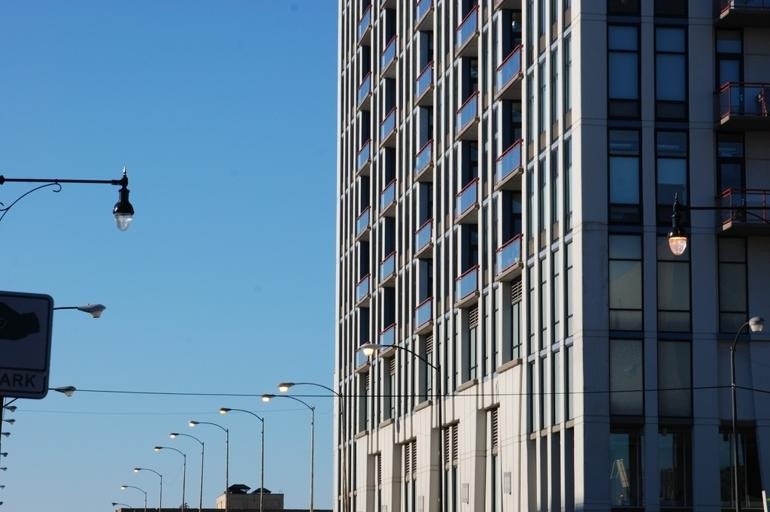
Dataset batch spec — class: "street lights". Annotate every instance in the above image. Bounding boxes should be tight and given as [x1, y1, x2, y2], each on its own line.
[112, 382, 345, 512]
[667, 192, 769, 257]
[47, 386, 76, 397]
[0, 165, 135, 232]
[730, 316, 765, 512]
[52, 303, 106, 319]
[359, 343, 442, 512]
